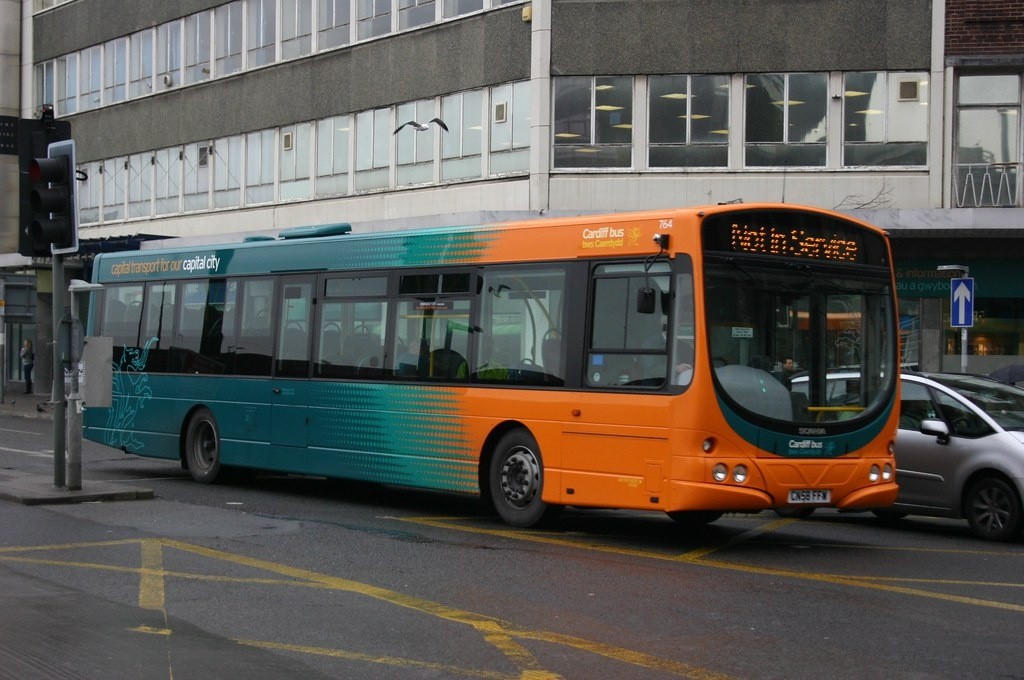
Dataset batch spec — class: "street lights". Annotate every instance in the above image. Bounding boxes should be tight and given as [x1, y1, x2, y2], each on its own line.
[936, 265, 972, 374]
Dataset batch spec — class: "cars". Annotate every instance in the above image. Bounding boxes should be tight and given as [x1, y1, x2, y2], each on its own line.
[773, 367, 1024, 546]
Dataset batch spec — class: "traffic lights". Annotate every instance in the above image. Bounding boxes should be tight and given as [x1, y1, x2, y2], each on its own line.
[26, 139, 81, 256]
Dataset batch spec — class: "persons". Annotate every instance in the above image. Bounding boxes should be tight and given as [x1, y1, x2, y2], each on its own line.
[20, 338, 34, 395]
[637, 313, 693, 384]
[781, 357, 794, 369]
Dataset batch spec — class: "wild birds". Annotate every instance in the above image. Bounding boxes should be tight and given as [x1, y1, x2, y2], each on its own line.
[395, 117, 449, 133]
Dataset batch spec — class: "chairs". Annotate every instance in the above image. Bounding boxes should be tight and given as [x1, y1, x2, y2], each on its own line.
[285, 320, 305, 352]
[123, 300, 141, 330]
[323, 322, 341, 356]
[107, 300, 127, 328]
[156, 302, 174, 331]
[344, 324, 372, 360]
[255, 308, 272, 337]
[541, 328, 561, 374]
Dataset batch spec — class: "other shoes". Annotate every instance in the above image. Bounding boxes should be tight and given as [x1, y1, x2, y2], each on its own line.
[22, 392, 32, 394]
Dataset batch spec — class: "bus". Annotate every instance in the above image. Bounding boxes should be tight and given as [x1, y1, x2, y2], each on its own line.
[80, 198, 905, 533]
